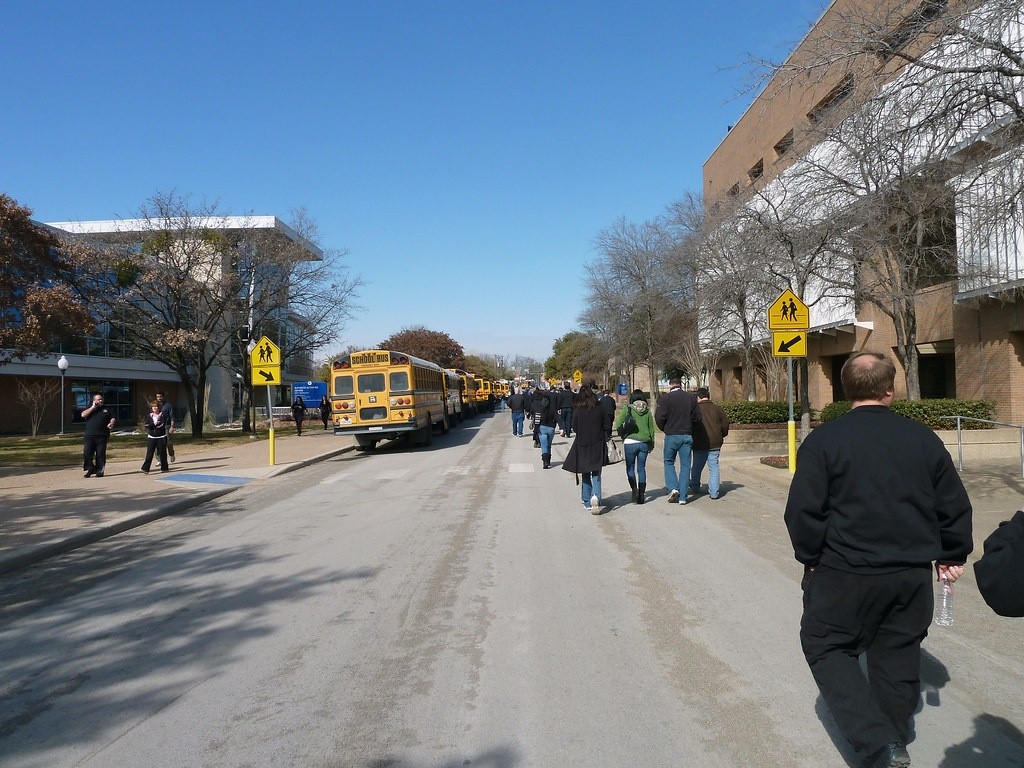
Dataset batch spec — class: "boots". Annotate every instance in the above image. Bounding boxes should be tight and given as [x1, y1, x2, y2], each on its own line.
[547, 453, 551, 466]
[637, 482, 646, 504]
[541, 453, 549, 470]
[629, 479, 637, 497]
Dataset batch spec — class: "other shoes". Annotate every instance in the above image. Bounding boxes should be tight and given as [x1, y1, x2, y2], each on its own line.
[534, 441, 541, 448]
[519, 435, 522, 436]
[96, 473, 104, 477]
[161, 469, 168, 473]
[84, 468, 95, 478]
[869, 742, 911, 768]
[140, 468, 149, 474]
[591, 495, 599, 515]
[155, 461, 162, 466]
[679, 500, 686, 504]
[667, 489, 679, 503]
[560, 429, 571, 437]
[512, 434, 517, 437]
[689, 479, 698, 493]
[171, 454, 175, 462]
[584, 504, 592, 510]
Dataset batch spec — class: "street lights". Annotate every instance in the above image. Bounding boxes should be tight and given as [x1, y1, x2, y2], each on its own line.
[57, 356, 68, 436]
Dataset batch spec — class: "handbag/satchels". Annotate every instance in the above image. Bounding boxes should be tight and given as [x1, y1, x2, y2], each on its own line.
[605, 439, 623, 464]
[617, 406, 636, 436]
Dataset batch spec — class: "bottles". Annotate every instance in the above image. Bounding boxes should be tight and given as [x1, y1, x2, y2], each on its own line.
[934, 574, 954, 627]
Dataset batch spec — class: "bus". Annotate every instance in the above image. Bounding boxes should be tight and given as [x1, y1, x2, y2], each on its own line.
[443, 368, 465, 421]
[331, 348, 451, 450]
[472, 373, 509, 412]
[446, 369, 480, 419]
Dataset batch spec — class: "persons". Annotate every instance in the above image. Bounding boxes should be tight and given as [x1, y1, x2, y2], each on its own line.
[690, 388, 729, 499]
[141, 391, 175, 473]
[319, 395, 331, 430]
[290, 397, 308, 437]
[507, 381, 617, 447]
[615, 389, 655, 504]
[784, 347, 977, 768]
[538, 396, 565, 469]
[81, 395, 116, 478]
[974, 510, 1024, 618]
[653, 378, 700, 504]
[561, 386, 614, 515]
